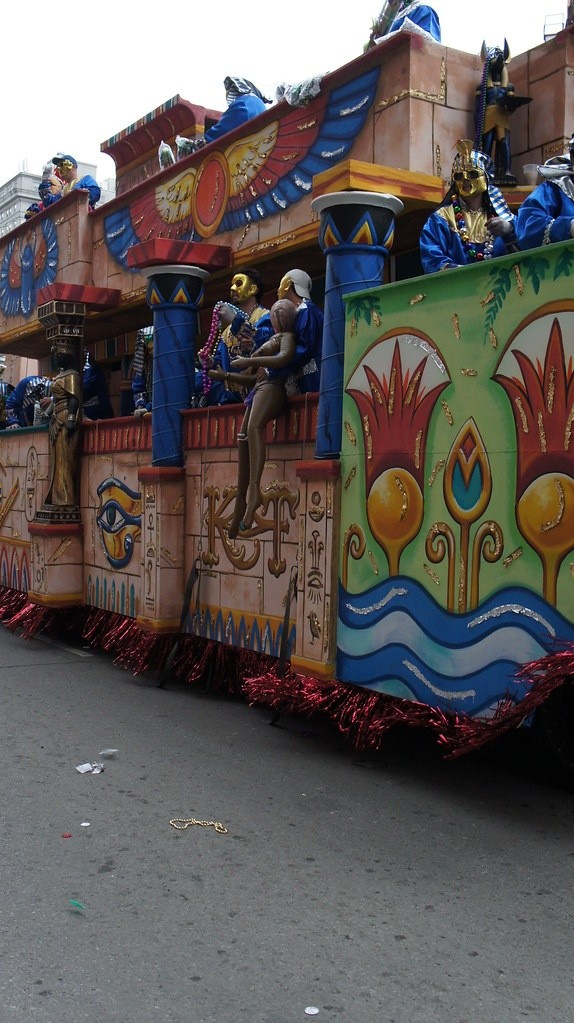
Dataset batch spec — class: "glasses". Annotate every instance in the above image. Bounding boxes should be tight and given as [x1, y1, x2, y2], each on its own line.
[454, 171, 484, 179]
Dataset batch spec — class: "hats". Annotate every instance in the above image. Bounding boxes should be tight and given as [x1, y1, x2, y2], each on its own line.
[435, 139, 514, 221]
[52, 154, 78, 168]
[288, 268, 312, 301]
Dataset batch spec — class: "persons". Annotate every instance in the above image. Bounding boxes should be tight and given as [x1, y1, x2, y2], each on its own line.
[40, 350, 114, 421]
[131, 325, 152, 417]
[513, 135, 574, 252]
[25, 178, 63, 220]
[0, 381, 15, 430]
[194, 268, 275, 409]
[240, 268, 319, 404]
[209, 298, 296, 540]
[40, 346, 82, 505]
[384, 0, 441, 43]
[38, 154, 101, 212]
[420, 148, 516, 275]
[181, 76, 273, 151]
[5, 376, 54, 429]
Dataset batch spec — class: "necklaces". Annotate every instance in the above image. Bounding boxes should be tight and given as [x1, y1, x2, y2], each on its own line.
[198, 301, 259, 394]
[451, 194, 495, 259]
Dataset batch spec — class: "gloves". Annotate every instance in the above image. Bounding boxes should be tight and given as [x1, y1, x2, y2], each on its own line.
[134, 408, 145, 425]
